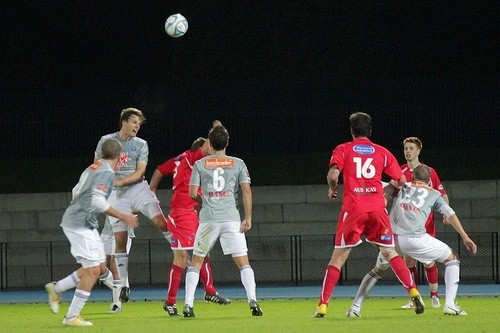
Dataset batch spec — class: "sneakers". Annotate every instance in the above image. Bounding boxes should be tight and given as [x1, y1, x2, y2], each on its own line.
[120, 286, 130, 303]
[402, 296, 425, 308]
[346, 308, 360, 317]
[249, 299, 263, 316]
[183, 304, 195, 317]
[409, 288, 424, 314]
[443, 305, 467, 315]
[163, 302, 179, 316]
[63, 315, 93, 326]
[112, 304, 120, 312]
[431, 295, 441, 308]
[45, 281, 62, 313]
[312, 304, 328, 317]
[204, 293, 231, 305]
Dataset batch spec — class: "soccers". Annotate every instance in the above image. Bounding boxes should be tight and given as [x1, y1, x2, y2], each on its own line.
[165, 13, 189, 38]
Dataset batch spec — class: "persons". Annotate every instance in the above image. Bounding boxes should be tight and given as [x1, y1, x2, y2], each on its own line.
[45, 137, 138, 326]
[347, 164, 477, 317]
[183, 125, 263, 317]
[383, 137, 449, 309]
[94, 108, 191, 303]
[150, 121, 232, 316]
[99, 213, 135, 314]
[312, 112, 426, 318]
